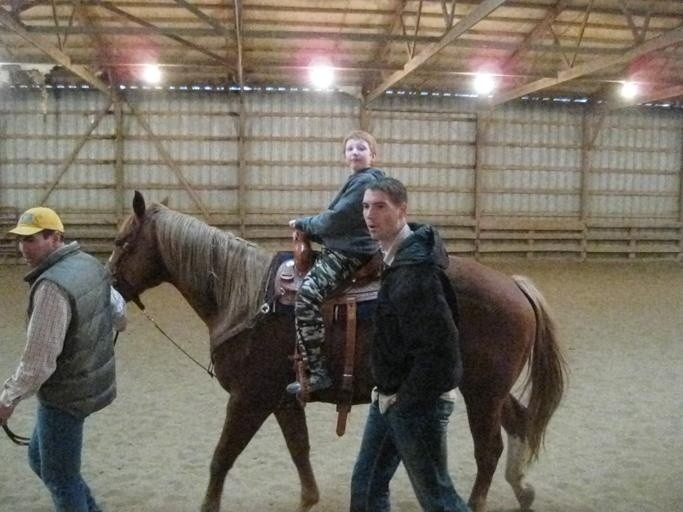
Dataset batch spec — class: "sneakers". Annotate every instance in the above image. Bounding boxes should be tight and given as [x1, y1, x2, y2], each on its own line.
[286, 373, 332, 395]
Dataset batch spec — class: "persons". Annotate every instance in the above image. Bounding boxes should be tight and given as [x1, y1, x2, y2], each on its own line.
[350, 176, 474, 512]
[1, 207, 127, 512]
[285, 128, 388, 394]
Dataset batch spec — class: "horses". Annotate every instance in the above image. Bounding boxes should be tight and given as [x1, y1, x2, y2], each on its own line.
[103, 190, 573, 512]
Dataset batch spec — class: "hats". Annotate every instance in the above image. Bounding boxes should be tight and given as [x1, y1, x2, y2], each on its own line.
[6, 207, 64, 239]
